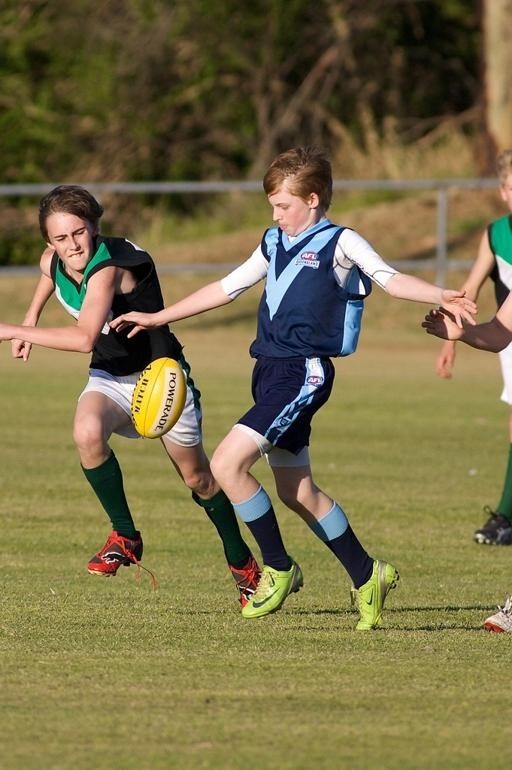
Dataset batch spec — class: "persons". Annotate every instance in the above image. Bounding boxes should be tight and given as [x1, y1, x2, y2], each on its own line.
[1, 185, 263, 609]
[107, 147, 480, 632]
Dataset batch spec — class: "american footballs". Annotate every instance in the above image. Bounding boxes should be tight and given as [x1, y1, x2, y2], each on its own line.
[129, 357, 187, 439]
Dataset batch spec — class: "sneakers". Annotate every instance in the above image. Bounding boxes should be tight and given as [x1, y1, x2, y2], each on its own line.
[350, 560, 400, 631]
[473, 513, 512, 544]
[228, 557, 303, 619]
[484, 598, 512, 633]
[87, 530, 143, 577]
